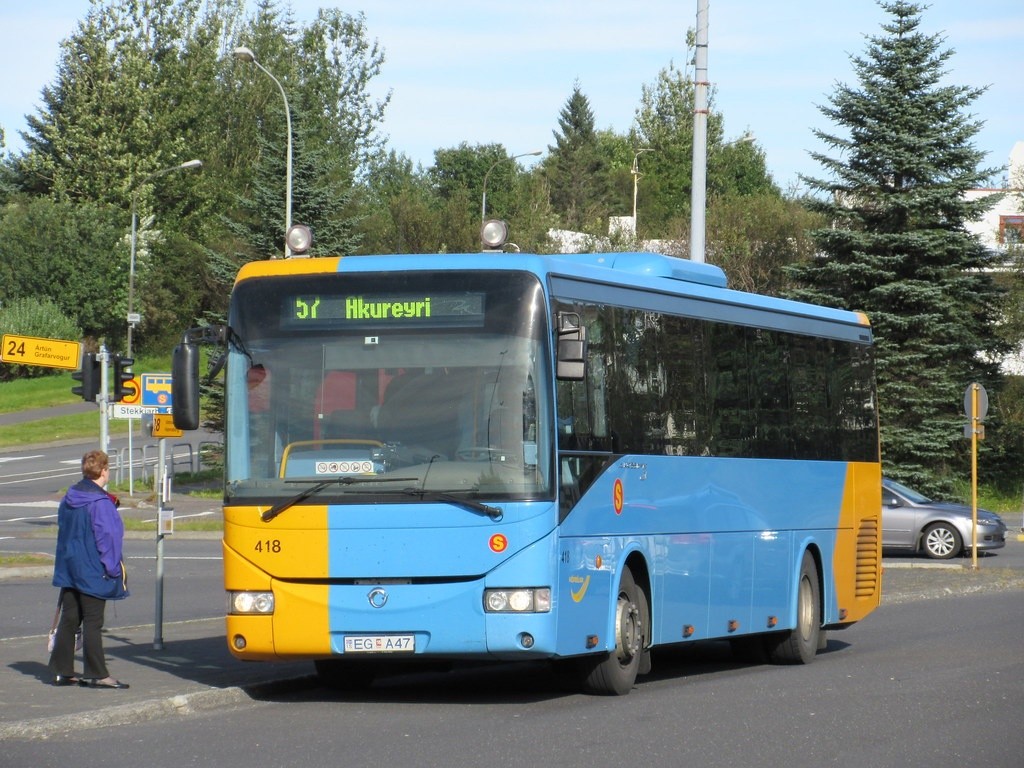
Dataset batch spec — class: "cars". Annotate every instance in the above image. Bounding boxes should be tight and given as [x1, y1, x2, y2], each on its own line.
[880, 475, 1009, 561]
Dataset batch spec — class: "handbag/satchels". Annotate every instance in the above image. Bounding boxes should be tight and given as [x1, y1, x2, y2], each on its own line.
[47, 627, 91, 653]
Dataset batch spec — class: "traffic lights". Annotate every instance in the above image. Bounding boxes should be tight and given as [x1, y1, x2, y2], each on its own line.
[71, 352, 101, 402]
[110, 353, 136, 402]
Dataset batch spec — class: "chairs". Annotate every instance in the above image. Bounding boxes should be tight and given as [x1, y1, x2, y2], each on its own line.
[318, 408, 379, 468]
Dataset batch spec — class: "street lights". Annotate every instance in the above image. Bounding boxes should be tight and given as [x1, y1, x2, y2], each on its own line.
[125, 158, 202, 375]
[631, 148, 656, 239]
[235, 46, 293, 259]
[480, 151, 544, 227]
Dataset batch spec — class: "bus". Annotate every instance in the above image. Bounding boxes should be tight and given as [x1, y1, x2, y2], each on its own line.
[173, 217, 886, 693]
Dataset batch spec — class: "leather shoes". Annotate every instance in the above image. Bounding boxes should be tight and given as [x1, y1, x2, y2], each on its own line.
[55, 675, 88, 687]
[91, 678, 129, 689]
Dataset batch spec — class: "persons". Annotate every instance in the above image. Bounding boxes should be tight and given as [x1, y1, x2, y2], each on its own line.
[47, 450, 131, 689]
